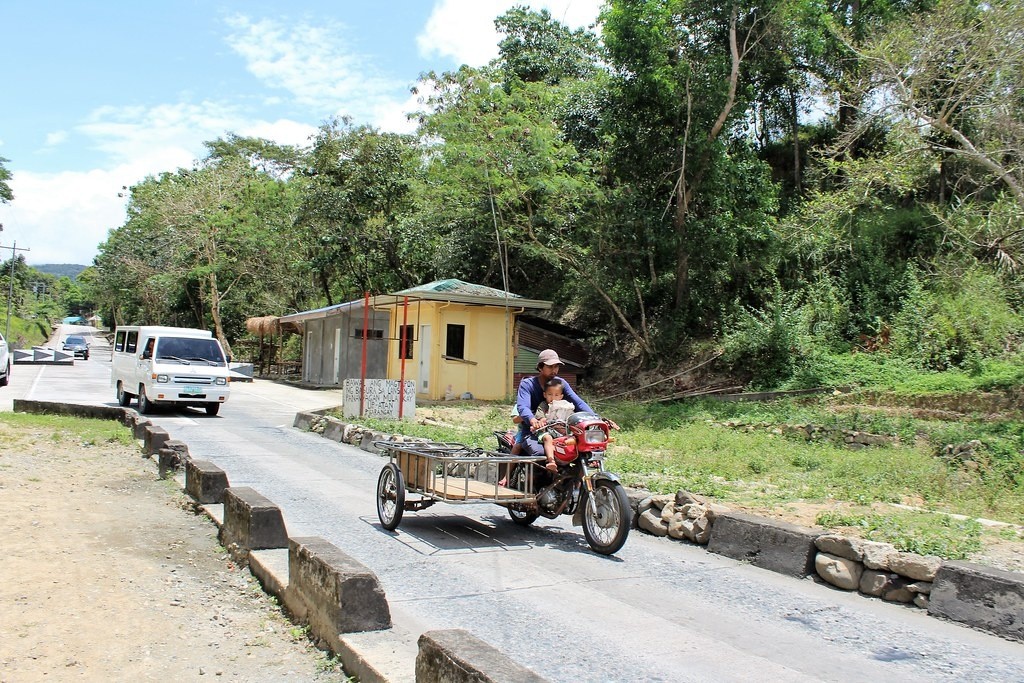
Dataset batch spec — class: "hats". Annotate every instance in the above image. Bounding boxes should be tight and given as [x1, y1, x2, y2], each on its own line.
[537, 349, 565, 366]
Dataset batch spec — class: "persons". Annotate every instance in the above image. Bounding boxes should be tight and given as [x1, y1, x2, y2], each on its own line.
[531, 378, 600, 473]
[517, 350, 621, 485]
[498, 376, 537, 487]
[139, 340, 153, 359]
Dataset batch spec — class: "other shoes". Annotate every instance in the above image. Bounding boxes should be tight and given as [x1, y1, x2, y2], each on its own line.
[545, 463, 558, 472]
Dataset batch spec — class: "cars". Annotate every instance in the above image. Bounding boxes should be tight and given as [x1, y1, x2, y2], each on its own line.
[61, 336, 90, 360]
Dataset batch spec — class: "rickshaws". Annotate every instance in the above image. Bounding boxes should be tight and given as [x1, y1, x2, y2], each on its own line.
[374, 409, 633, 557]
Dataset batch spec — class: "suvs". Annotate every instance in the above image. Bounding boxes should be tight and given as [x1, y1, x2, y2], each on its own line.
[0, 332, 11, 386]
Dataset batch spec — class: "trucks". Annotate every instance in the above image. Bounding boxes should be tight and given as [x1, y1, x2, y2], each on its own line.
[110, 324, 232, 417]
